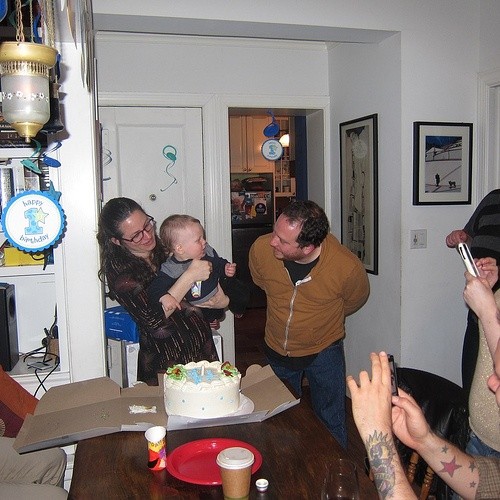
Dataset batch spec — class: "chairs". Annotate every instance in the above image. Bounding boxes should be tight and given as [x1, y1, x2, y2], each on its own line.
[366, 368, 468, 500]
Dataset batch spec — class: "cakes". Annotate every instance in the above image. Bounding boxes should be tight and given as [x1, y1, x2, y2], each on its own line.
[163, 360, 241, 418]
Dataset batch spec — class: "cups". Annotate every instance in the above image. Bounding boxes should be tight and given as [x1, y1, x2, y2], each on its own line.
[216, 447, 255, 499]
[144, 426, 167, 470]
[321, 458, 360, 500]
[46, 337, 59, 361]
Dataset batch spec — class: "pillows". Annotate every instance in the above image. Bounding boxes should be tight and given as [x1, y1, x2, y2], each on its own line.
[0, 365, 40, 437]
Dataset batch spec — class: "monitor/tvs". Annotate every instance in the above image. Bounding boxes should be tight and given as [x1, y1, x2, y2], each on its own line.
[229, 172, 274, 227]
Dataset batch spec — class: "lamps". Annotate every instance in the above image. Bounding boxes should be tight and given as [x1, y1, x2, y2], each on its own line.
[0, 0, 58, 143]
[279, 129, 290, 147]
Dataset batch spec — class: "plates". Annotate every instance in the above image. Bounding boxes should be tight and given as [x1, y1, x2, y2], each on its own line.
[165, 438, 263, 486]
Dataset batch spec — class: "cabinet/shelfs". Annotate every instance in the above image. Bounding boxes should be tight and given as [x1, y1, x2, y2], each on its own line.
[231, 115, 274, 174]
[0, 25, 71, 382]
[275, 117, 290, 193]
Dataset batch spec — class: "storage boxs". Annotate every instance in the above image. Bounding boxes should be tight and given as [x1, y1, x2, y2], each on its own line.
[104, 305, 138, 343]
[3, 247, 44, 266]
[232, 191, 272, 215]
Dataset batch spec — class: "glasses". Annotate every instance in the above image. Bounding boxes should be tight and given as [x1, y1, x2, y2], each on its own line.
[120, 214, 155, 244]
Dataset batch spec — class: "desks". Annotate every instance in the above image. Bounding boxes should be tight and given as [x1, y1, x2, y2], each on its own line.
[65, 379, 379, 500]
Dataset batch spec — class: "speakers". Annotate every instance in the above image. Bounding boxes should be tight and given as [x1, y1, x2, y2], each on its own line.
[0, 282, 19, 371]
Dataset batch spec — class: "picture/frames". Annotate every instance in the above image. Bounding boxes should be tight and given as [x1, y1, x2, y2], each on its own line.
[413, 121, 471, 205]
[339, 112, 378, 275]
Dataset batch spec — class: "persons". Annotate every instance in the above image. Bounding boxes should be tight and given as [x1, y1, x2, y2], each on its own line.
[232, 177, 268, 217]
[347, 188, 500, 500]
[0, 437, 69, 500]
[149, 214, 245, 328]
[248, 200, 370, 451]
[97, 197, 249, 387]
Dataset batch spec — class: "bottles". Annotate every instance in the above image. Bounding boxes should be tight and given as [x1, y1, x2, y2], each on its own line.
[252, 478, 276, 500]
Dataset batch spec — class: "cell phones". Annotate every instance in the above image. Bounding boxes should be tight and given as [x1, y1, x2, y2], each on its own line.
[386, 353, 399, 407]
[458, 242, 481, 277]
[27, 362, 53, 370]
